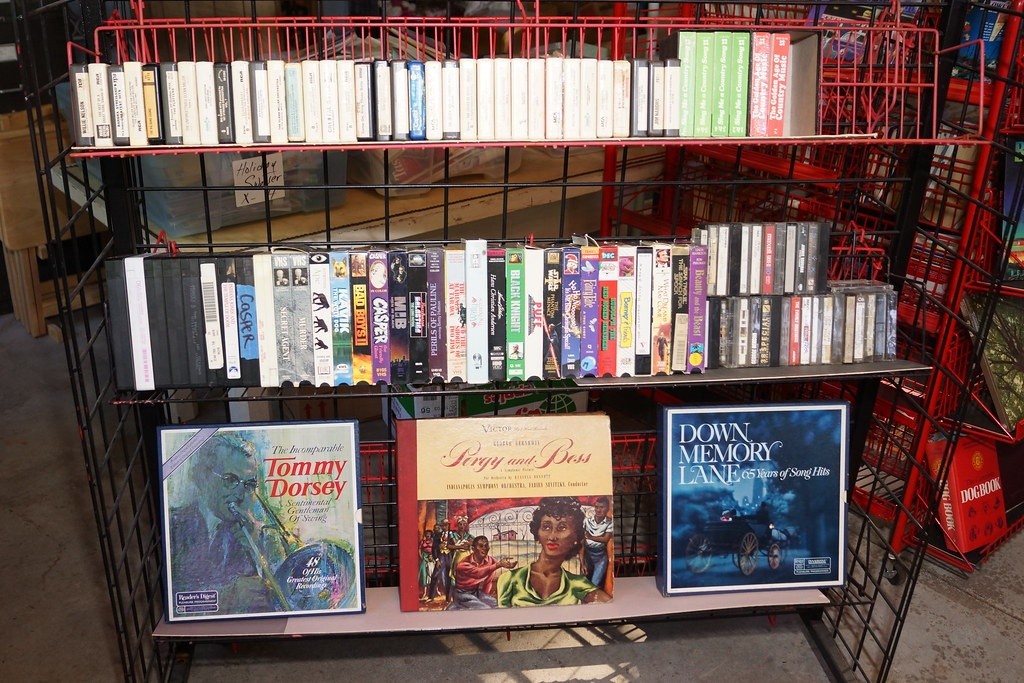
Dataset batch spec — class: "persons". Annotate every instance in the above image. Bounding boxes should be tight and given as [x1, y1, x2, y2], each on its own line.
[656, 249, 671, 267]
[509, 344, 522, 359]
[656, 331, 669, 362]
[545, 324, 561, 365]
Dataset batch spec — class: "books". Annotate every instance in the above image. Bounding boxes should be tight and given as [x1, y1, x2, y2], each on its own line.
[68, 0, 818, 148]
[102, 152, 899, 391]
[819, 0, 1024, 553]
[158, 403, 851, 624]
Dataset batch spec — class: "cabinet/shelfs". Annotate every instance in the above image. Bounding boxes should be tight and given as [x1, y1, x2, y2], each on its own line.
[8, 0, 1024, 681]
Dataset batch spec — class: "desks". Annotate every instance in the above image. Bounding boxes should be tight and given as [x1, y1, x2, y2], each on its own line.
[0, 101, 111, 338]
